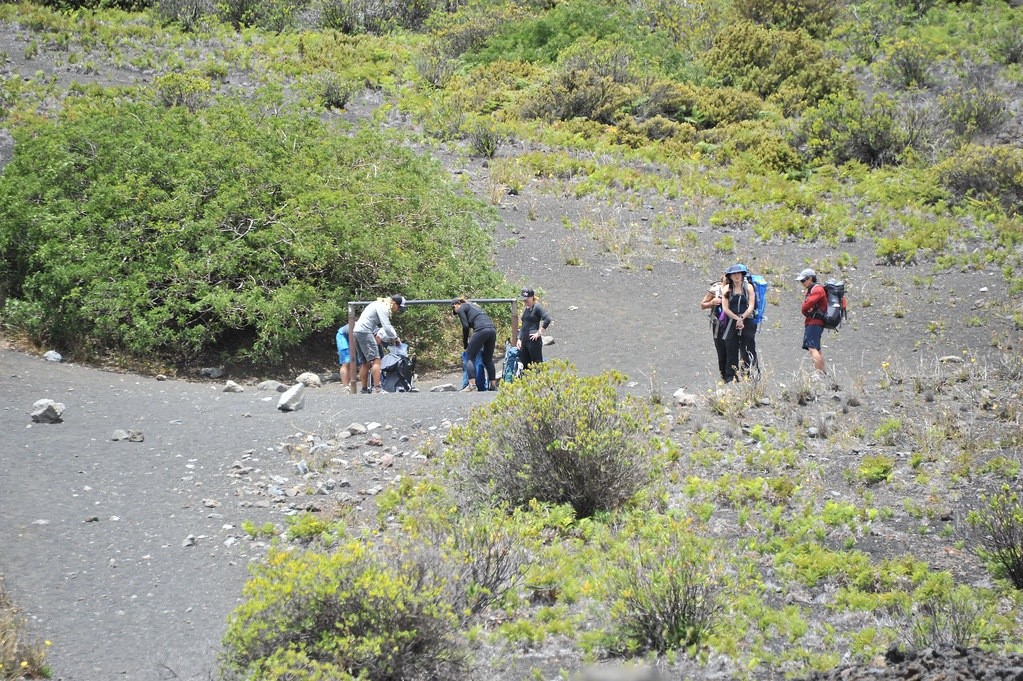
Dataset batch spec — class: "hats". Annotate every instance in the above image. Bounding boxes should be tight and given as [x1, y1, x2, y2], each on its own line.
[794, 268, 815, 280]
[392, 295, 407, 308]
[519, 288, 535, 301]
[451, 298, 461, 315]
[725, 265, 746, 276]
[376, 327, 389, 343]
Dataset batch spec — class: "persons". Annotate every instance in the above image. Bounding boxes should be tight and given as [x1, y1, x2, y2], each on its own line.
[450, 298, 496, 392]
[795, 268, 827, 372]
[352, 294, 407, 394]
[516, 288, 553, 369]
[336, 321, 393, 395]
[700, 264, 760, 385]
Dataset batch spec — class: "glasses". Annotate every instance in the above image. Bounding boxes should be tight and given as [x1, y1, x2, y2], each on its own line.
[800, 277, 811, 282]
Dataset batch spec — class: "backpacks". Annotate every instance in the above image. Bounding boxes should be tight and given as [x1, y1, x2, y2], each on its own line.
[730, 275, 767, 323]
[378, 343, 415, 393]
[810, 277, 847, 328]
[501, 347, 525, 385]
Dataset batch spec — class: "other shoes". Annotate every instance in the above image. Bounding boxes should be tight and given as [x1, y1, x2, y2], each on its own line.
[372, 388, 389, 394]
[460, 383, 478, 392]
[489, 381, 497, 391]
[343, 387, 351, 394]
[361, 389, 371, 394]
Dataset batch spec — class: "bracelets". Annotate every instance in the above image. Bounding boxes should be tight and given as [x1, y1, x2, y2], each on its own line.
[395, 336, 398, 339]
